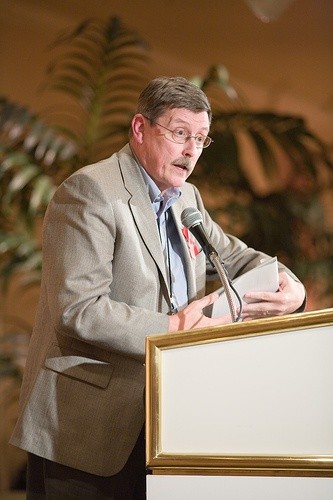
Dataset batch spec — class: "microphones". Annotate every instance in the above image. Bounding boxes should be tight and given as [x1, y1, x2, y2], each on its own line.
[180, 207, 217, 258]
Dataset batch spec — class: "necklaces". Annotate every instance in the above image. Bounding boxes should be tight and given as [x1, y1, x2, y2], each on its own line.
[165, 211, 179, 314]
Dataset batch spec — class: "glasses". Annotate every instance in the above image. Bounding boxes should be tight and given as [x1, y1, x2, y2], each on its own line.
[145, 117, 213, 148]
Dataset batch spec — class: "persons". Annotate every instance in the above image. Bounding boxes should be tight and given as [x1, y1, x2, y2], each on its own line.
[8, 76, 308, 500]
